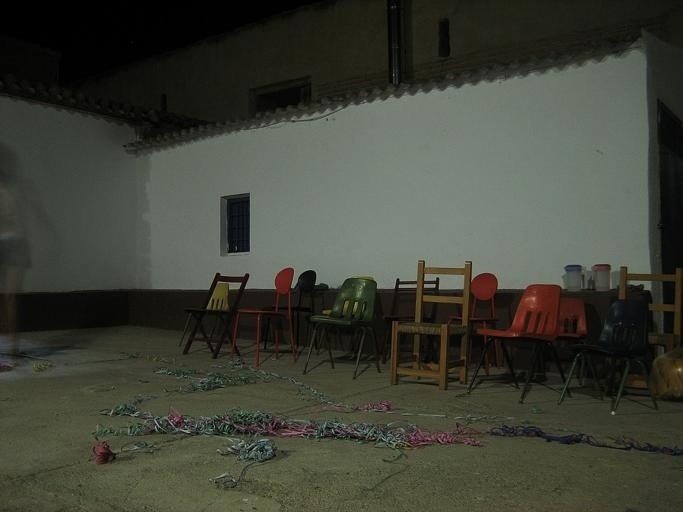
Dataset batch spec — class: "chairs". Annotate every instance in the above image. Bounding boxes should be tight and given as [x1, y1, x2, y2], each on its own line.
[262, 270, 441, 365]
[555, 300, 659, 414]
[302, 276, 381, 381]
[180, 272, 250, 361]
[465, 281, 562, 404]
[447, 271, 606, 387]
[610, 265, 682, 394]
[228, 265, 296, 366]
[387, 258, 474, 389]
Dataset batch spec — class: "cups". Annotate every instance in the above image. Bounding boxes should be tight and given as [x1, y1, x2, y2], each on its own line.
[562, 264, 612, 292]
